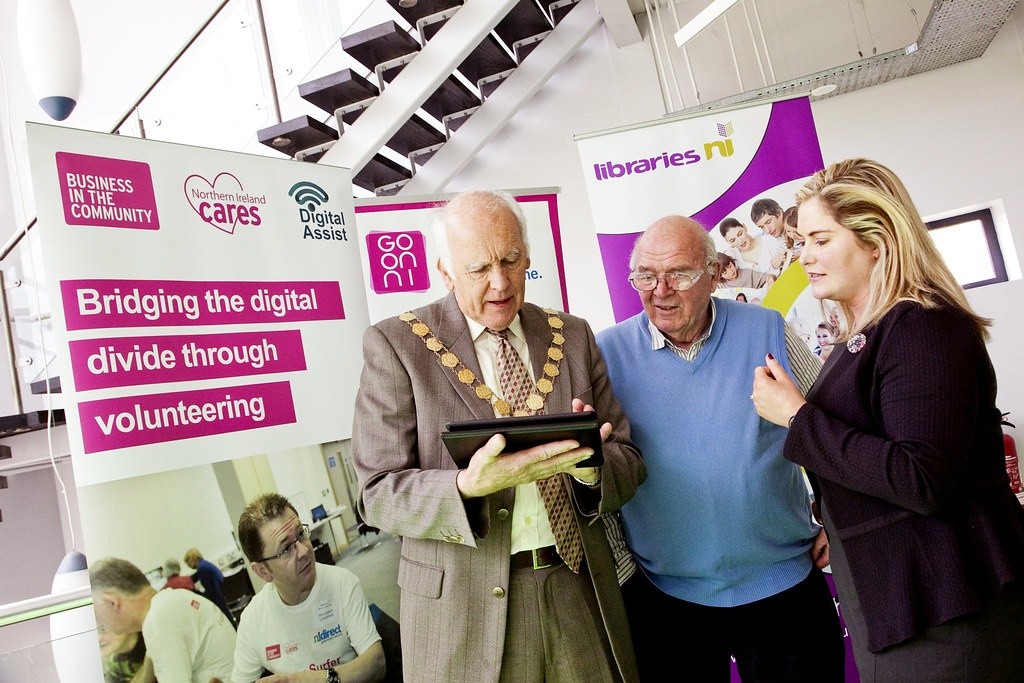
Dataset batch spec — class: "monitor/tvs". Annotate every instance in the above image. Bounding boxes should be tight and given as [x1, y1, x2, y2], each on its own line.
[311, 504, 327, 523]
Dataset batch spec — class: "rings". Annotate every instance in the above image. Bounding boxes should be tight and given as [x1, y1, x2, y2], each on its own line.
[750, 394, 754, 398]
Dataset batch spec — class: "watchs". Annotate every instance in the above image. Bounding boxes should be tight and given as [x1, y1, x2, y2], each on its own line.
[325, 668, 339, 683]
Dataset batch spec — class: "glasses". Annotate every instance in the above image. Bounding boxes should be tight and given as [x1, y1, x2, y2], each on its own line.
[628, 262, 711, 293]
[255, 524, 311, 563]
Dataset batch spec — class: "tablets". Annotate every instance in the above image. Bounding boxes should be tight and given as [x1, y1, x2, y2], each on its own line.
[445, 412, 599, 432]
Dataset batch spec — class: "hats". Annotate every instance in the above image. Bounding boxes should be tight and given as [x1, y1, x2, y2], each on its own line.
[160, 560, 181, 578]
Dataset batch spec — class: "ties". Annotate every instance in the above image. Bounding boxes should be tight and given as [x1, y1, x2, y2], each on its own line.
[486, 327, 585, 574]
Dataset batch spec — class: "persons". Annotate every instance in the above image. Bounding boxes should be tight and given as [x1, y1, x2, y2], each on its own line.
[351, 193, 648, 683]
[714, 198, 849, 363]
[594, 215, 846, 683]
[750, 159, 1024, 683]
[88, 490, 388, 683]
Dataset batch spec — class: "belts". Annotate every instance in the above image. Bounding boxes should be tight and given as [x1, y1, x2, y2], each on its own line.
[509, 546, 565, 571]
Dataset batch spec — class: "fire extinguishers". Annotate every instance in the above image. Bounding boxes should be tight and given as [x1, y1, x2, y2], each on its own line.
[995, 406, 1024, 494]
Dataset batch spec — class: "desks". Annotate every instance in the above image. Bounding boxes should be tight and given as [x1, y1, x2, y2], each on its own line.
[301, 510, 351, 556]
[192, 561, 250, 620]
